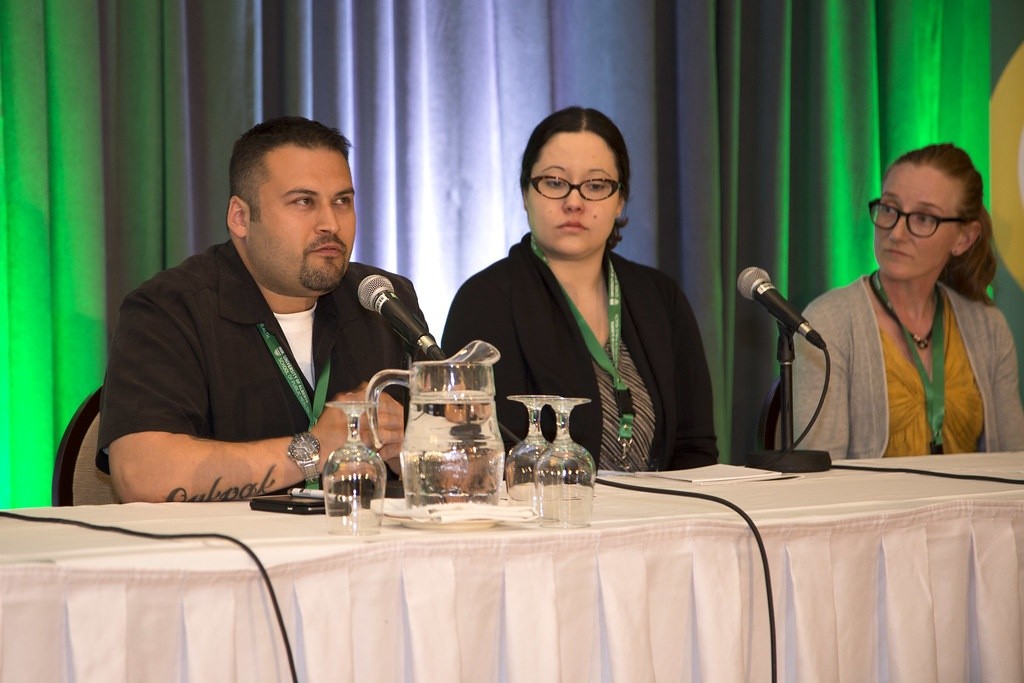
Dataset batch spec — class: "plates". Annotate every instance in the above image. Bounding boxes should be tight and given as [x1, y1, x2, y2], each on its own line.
[385, 514, 499, 531]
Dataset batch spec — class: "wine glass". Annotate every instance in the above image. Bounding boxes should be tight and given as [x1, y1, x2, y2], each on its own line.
[507, 395, 560, 504]
[533, 397, 597, 530]
[324, 400, 387, 535]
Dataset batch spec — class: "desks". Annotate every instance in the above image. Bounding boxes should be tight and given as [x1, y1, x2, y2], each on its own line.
[0, 451, 1024, 683]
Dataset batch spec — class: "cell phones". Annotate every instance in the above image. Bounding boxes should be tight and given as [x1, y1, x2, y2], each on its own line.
[249, 497, 327, 514]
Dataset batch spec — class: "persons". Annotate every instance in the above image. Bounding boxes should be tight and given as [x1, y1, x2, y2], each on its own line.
[442, 105, 718, 484]
[774, 141, 1023, 459]
[95, 114, 446, 504]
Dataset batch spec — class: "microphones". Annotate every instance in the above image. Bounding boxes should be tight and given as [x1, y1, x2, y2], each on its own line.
[356, 274, 438, 353]
[737, 267, 829, 348]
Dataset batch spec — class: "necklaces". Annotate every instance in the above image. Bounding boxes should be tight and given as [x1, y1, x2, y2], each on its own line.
[867, 270, 933, 350]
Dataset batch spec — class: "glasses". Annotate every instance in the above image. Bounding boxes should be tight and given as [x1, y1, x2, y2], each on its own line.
[528, 174, 620, 203]
[868, 198, 964, 238]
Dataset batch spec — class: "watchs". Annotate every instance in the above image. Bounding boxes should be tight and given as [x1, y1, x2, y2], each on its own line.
[287, 431, 321, 484]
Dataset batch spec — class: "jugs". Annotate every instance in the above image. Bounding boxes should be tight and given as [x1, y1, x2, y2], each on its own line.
[365, 340, 506, 509]
